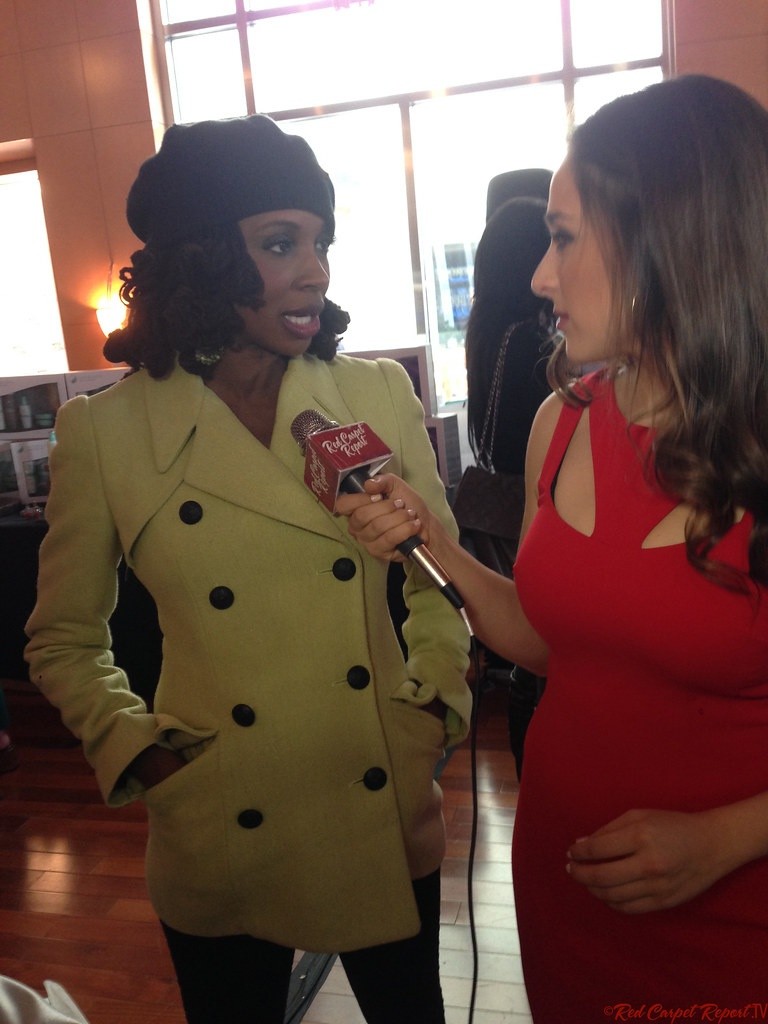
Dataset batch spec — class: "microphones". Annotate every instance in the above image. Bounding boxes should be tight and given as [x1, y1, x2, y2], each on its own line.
[292, 410, 464, 612]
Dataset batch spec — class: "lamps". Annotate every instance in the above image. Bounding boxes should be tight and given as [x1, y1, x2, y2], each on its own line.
[97, 291, 128, 339]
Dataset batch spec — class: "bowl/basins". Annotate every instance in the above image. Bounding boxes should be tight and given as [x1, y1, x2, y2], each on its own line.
[35, 418, 53, 428]
[35, 412, 53, 420]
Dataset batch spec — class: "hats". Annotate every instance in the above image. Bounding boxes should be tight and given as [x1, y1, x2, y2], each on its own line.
[127, 114, 336, 240]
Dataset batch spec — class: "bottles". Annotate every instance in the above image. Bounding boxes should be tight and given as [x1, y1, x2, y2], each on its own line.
[24, 461, 36, 496]
[19, 500, 33, 520]
[47, 430, 59, 475]
[18, 395, 32, 429]
[4, 395, 19, 431]
[29, 499, 44, 521]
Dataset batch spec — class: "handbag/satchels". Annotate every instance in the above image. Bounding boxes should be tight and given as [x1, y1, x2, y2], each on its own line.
[452, 465, 523, 544]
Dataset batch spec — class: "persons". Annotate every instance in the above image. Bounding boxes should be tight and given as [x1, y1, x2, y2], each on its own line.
[330, 73, 768, 1022]
[24, 116, 472, 1024]
[464, 198, 557, 790]
